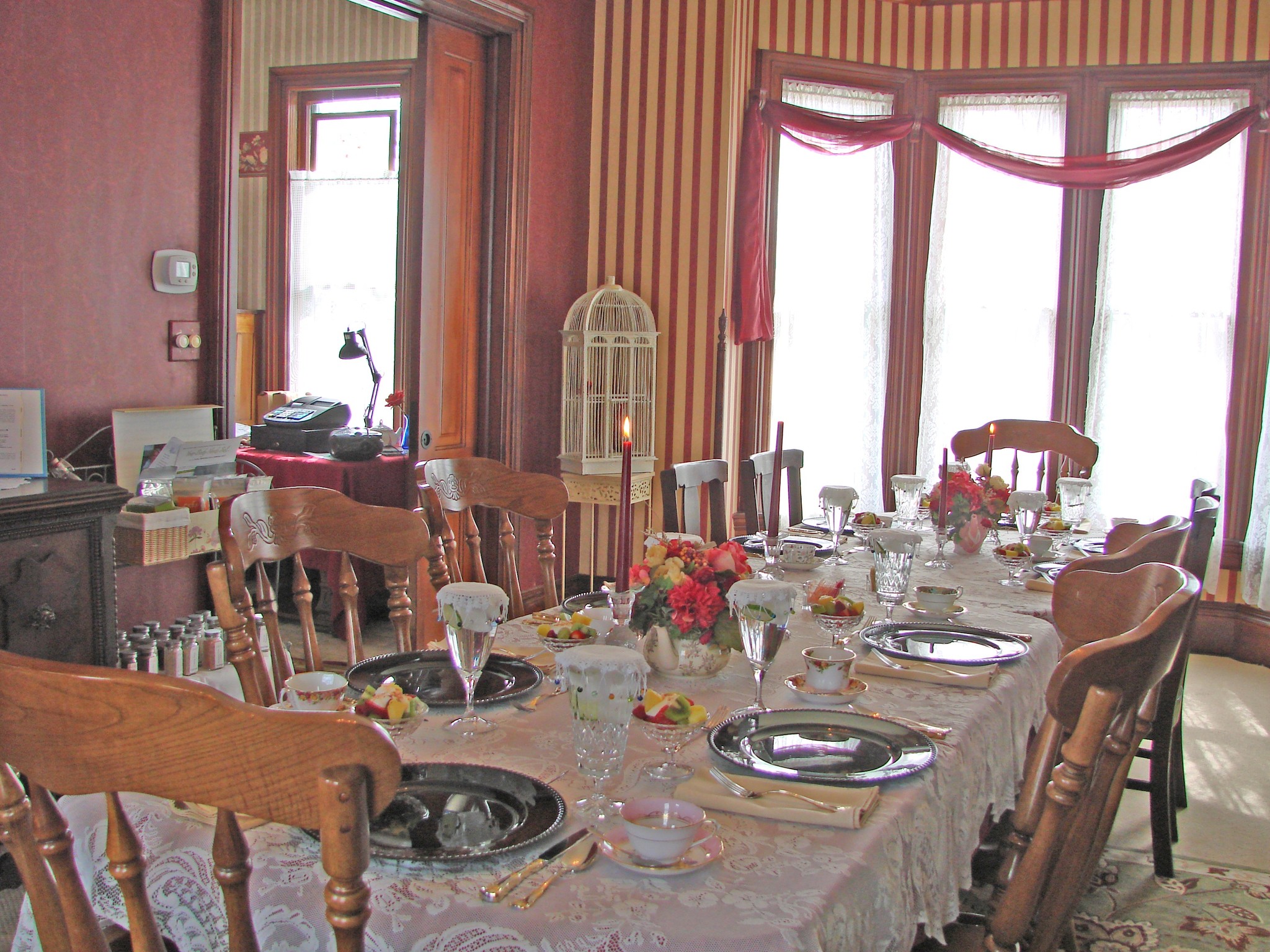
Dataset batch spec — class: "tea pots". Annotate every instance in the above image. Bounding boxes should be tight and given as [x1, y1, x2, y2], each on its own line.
[370, 420, 403, 447]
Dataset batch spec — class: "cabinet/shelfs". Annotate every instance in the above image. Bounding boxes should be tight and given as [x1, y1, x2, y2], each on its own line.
[0, 475, 131, 669]
[47, 424, 295, 703]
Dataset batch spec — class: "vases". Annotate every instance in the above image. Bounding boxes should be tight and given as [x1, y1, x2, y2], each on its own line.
[641, 622, 728, 679]
[401, 415, 413, 449]
[952, 513, 990, 556]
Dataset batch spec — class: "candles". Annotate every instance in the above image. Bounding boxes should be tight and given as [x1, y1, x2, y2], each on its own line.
[767, 422, 784, 537]
[938, 448, 948, 535]
[614, 417, 635, 591]
[984, 422, 995, 477]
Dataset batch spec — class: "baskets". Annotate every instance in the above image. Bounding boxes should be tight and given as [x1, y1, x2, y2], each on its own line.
[117, 507, 191, 565]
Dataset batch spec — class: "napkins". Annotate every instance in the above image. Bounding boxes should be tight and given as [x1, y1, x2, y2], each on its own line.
[855, 650, 1002, 689]
[429, 636, 563, 675]
[675, 768, 881, 829]
[779, 530, 848, 544]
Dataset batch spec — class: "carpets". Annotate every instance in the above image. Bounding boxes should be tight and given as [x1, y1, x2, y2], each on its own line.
[1059, 846, 1270, 952]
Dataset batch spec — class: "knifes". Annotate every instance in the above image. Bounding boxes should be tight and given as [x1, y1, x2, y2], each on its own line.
[479, 826, 597, 902]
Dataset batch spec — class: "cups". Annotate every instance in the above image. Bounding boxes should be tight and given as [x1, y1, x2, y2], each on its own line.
[1058, 478, 1090, 527]
[801, 646, 857, 693]
[278, 671, 348, 711]
[1022, 536, 1052, 557]
[1111, 518, 1139, 530]
[619, 797, 718, 867]
[779, 543, 816, 564]
[913, 586, 963, 611]
[877, 515, 893, 529]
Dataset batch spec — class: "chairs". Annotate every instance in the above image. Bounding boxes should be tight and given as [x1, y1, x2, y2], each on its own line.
[0, 455, 570, 952]
[660, 449, 804, 544]
[914, 418, 1222, 952]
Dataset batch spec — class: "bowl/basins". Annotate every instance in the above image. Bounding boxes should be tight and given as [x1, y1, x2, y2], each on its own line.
[372, 701, 430, 737]
[1041, 511, 1061, 523]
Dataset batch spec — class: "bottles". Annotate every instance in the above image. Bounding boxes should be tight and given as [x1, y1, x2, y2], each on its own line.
[49, 458, 81, 480]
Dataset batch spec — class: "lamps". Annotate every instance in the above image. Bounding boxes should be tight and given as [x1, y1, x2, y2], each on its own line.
[337, 325, 383, 429]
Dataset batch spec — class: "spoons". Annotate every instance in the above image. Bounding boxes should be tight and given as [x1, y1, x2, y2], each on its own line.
[848, 703, 952, 734]
[947, 617, 1032, 642]
[511, 842, 599, 909]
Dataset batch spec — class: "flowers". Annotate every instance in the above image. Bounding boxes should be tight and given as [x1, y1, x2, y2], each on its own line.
[628, 527, 752, 653]
[384, 390, 406, 416]
[922, 459, 1012, 546]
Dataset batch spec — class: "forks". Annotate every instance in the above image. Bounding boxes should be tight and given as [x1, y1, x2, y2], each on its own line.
[492, 647, 547, 661]
[668, 705, 729, 753]
[1040, 572, 1054, 583]
[838, 616, 877, 645]
[871, 647, 968, 676]
[709, 766, 840, 812]
[511, 690, 568, 712]
[1078, 547, 1091, 557]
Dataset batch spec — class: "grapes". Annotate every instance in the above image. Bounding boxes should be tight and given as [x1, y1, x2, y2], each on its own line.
[558, 627, 570, 639]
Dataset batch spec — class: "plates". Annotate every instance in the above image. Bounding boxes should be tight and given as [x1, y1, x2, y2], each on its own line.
[1102, 528, 1111, 535]
[784, 673, 869, 704]
[598, 825, 724, 875]
[268, 696, 356, 711]
[853, 532, 864, 541]
[778, 557, 826, 571]
[902, 601, 968, 620]
[1033, 552, 1062, 564]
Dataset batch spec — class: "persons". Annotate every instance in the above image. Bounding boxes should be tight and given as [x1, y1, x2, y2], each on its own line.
[141, 444, 166, 470]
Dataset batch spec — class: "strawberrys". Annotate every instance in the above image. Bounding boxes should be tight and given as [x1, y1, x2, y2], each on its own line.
[633, 698, 695, 730]
[832, 602, 858, 627]
[999, 547, 1030, 563]
[546, 630, 585, 652]
[853, 512, 881, 524]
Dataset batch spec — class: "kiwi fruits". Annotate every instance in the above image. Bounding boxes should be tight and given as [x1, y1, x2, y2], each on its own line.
[354, 684, 416, 721]
[570, 622, 596, 637]
[1014, 543, 1024, 554]
[811, 603, 826, 613]
[664, 695, 691, 722]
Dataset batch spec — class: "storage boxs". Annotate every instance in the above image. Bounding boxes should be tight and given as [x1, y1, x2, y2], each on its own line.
[110, 404, 226, 556]
[257, 391, 293, 425]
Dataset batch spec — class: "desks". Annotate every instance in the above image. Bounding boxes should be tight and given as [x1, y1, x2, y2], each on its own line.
[9, 521, 1105, 952]
[233, 445, 409, 640]
[560, 470, 656, 605]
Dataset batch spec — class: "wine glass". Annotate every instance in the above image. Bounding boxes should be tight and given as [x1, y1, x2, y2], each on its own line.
[1037, 526, 1073, 552]
[1015, 492, 1044, 542]
[439, 473, 930, 816]
[994, 552, 1034, 586]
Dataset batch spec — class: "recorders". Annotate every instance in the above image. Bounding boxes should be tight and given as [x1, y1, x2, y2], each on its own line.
[328, 429, 384, 460]
